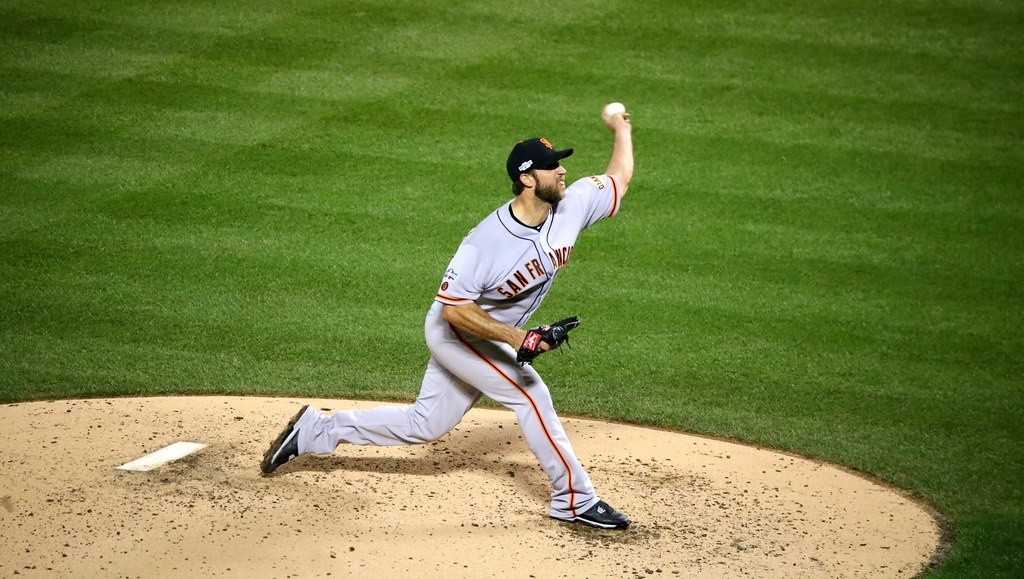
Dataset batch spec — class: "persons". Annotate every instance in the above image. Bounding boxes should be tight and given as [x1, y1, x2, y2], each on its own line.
[260, 102, 634, 529]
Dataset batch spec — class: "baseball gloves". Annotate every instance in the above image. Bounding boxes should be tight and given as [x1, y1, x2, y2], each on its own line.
[516, 316, 580, 367]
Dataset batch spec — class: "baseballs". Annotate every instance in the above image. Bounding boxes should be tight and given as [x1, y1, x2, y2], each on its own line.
[603, 102, 625, 117]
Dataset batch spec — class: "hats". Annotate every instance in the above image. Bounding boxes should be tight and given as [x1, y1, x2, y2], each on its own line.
[507, 137, 574, 182]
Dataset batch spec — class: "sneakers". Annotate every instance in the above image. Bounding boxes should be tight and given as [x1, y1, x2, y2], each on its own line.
[549, 500, 631, 530]
[260, 405, 311, 474]
